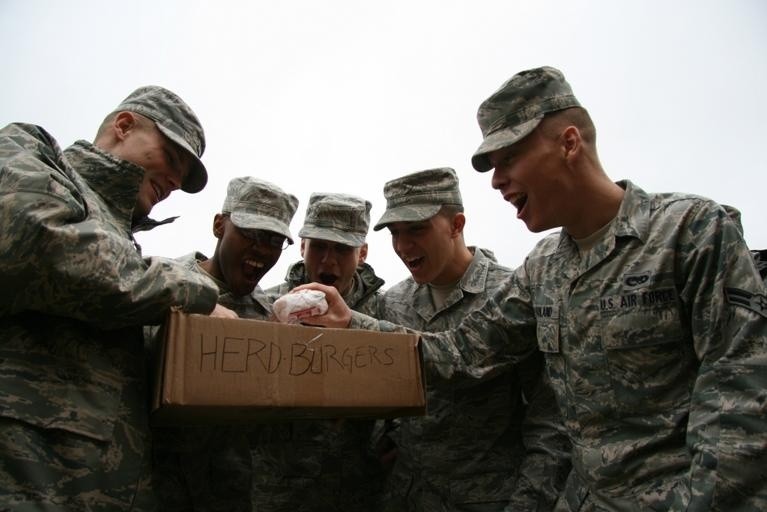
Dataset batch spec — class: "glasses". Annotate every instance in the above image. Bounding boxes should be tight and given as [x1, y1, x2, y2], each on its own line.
[238, 225, 289, 250]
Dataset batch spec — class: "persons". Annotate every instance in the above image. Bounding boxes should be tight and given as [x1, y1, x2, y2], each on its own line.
[1, 85, 241, 512]
[342, 165, 575, 511]
[290, 65, 766, 512]
[258, 191, 398, 512]
[136, 173, 309, 512]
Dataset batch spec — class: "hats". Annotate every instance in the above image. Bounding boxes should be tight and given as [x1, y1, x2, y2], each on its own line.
[223, 177, 300, 246]
[473, 68, 581, 172]
[300, 189, 373, 248]
[117, 86, 208, 194]
[374, 167, 462, 230]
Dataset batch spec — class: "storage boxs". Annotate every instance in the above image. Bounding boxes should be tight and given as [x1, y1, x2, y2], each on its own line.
[149, 309, 426, 422]
